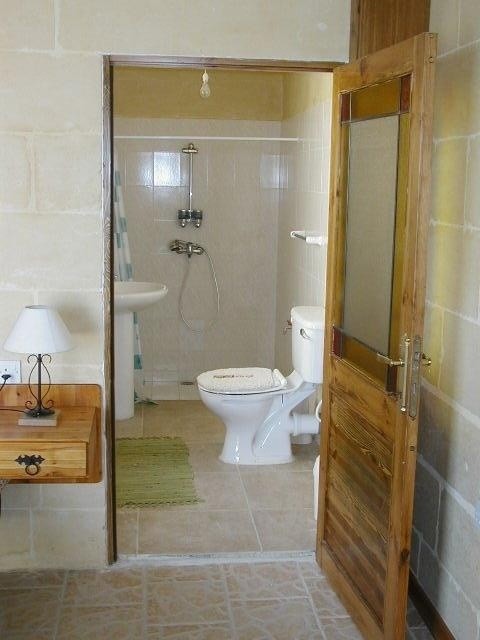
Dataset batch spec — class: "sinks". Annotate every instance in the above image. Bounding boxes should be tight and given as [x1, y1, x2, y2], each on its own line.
[113, 279, 167, 311]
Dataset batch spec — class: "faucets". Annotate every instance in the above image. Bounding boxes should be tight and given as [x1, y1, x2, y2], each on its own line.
[187, 243, 192, 257]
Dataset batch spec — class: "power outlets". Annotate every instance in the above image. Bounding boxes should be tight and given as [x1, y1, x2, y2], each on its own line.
[0, 361, 22, 386]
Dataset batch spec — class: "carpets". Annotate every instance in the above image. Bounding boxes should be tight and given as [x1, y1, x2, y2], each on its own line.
[115, 436, 201, 509]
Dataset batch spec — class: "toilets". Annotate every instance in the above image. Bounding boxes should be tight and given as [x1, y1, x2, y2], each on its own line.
[197, 306, 324, 465]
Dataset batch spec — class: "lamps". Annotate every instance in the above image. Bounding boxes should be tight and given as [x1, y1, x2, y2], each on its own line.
[4, 304, 75, 428]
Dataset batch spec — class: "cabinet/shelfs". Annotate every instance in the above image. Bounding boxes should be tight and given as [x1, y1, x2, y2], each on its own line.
[1, 405, 96, 481]
